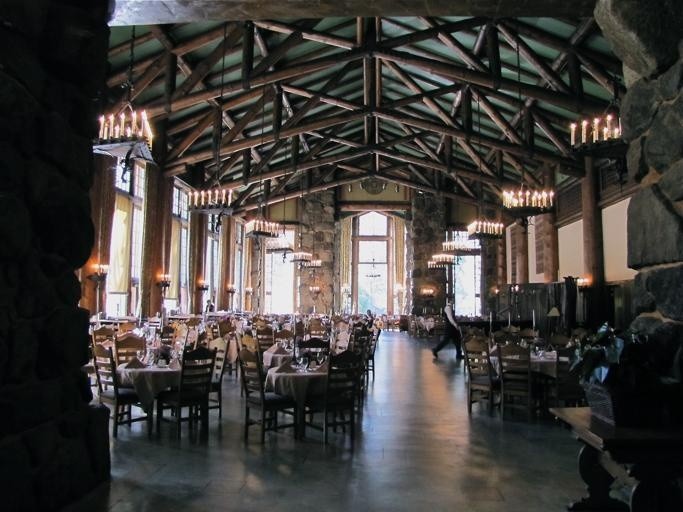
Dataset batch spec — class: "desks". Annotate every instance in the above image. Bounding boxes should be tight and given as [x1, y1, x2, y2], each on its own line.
[547, 403, 683, 511]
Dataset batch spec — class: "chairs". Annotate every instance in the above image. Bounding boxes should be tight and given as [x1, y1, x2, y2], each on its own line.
[381, 307, 496, 341]
[464, 307, 615, 420]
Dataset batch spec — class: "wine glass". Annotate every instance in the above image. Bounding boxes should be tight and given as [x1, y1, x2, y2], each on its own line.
[300, 357, 308, 373]
[316, 352, 324, 368]
[146, 348, 180, 369]
[518, 337, 558, 359]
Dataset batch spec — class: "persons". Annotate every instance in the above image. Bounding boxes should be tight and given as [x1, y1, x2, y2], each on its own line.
[429, 294, 466, 361]
[621, 293, 664, 341]
[363, 309, 373, 322]
[206, 300, 214, 312]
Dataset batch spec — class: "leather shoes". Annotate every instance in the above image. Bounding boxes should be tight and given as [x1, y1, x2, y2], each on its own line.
[432, 348, 438, 357]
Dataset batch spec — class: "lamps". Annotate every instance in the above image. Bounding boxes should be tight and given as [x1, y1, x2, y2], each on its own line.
[348, 180, 425, 194]
[94, 25, 158, 186]
[364, 256, 382, 280]
[186, 23, 235, 233]
[155, 273, 171, 298]
[576, 276, 590, 295]
[570, 54, 630, 158]
[265, 139, 322, 274]
[226, 283, 236, 297]
[499, 14, 557, 219]
[466, 76, 504, 240]
[86, 264, 110, 290]
[196, 280, 209, 296]
[427, 134, 481, 271]
[244, 19, 281, 240]
[308, 286, 321, 300]
